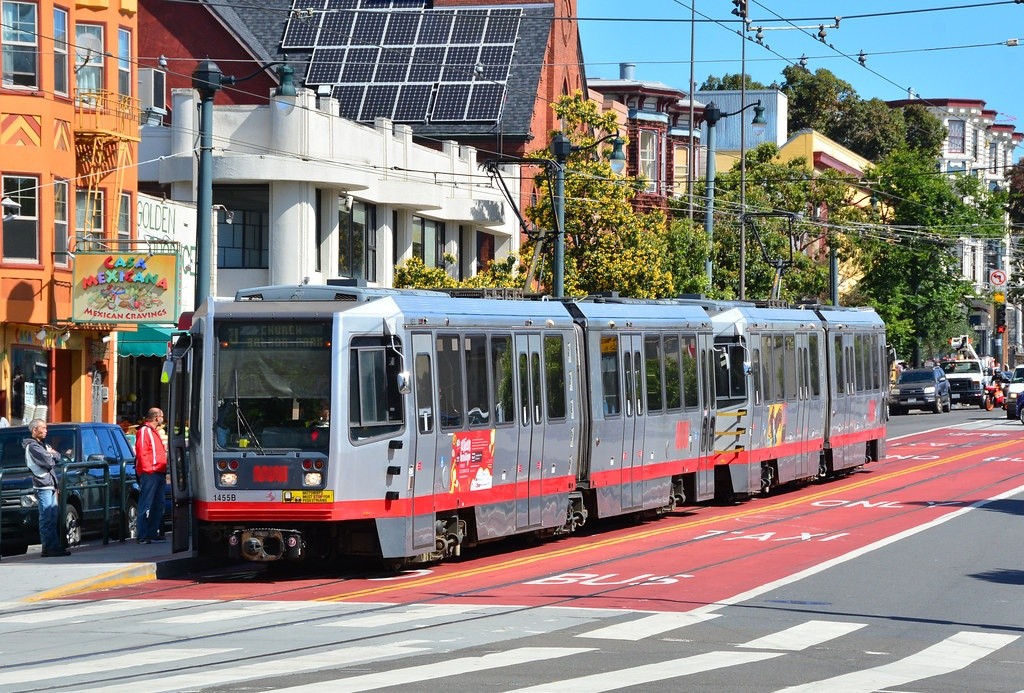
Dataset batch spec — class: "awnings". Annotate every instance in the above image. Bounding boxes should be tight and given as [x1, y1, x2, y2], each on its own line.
[117, 324, 187, 357]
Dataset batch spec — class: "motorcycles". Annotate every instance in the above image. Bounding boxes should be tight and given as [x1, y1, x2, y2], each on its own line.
[984, 373, 1010, 411]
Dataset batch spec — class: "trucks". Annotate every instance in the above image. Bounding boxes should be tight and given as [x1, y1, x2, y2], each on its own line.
[937, 359, 994, 410]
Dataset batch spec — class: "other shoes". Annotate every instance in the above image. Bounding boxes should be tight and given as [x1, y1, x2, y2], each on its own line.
[137, 538, 152, 544]
[151, 536, 166, 542]
[40, 550, 71, 557]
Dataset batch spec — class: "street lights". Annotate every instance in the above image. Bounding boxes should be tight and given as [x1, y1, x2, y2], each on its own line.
[551, 128, 627, 298]
[191, 50, 298, 312]
[987, 237, 1003, 369]
[828, 192, 879, 305]
[702, 96, 768, 299]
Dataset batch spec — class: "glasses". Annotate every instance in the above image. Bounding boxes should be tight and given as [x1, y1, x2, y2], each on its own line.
[159, 416, 164, 418]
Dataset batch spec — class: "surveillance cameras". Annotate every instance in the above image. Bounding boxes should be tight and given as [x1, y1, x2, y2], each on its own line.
[344, 196, 354, 211]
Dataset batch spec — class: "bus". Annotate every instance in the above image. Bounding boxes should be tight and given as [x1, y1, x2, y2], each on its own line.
[159, 277, 890, 572]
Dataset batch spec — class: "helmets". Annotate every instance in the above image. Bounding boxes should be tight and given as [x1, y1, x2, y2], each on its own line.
[995, 368, 1001, 373]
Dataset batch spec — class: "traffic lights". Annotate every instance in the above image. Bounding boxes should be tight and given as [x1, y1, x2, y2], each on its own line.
[994, 304, 1006, 336]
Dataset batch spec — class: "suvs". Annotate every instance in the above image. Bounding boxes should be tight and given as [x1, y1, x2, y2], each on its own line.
[889, 365, 952, 415]
[0, 422, 137, 562]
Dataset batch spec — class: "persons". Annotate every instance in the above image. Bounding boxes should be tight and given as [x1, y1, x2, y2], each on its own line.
[1003, 364, 1013, 379]
[317, 398, 360, 428]
[944, 355, 949, 361]
[991, 369, 1010, 397]
[135, 408, 168, 545]
[216, 408, 230, 447]
[23, 418, 71, 557]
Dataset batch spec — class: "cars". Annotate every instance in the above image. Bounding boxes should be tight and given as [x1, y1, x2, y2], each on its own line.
[125, 434, 193, 535]
[983, 363, 1024, 425]
